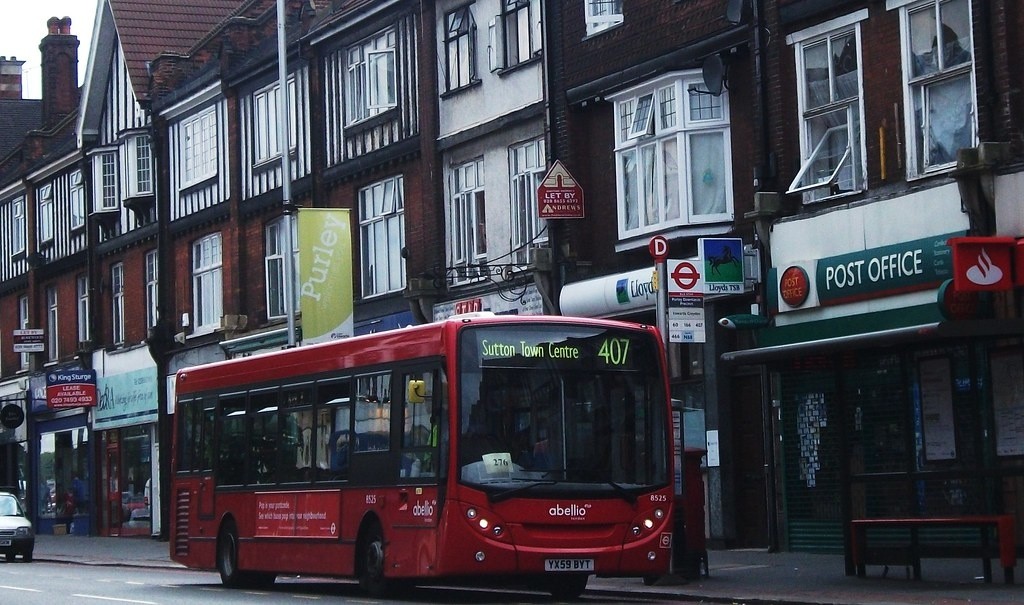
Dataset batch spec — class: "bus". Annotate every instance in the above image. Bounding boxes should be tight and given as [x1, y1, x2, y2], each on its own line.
[170, 310, 683, 601]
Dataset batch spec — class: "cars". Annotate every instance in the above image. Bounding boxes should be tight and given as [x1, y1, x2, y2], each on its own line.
[0, 492, 35, 563]
